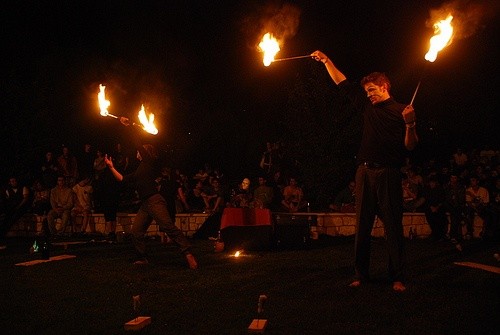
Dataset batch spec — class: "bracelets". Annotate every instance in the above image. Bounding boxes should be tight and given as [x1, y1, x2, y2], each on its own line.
[406, 122, 416, 128]
[323, 57, 328, 64]
[404, 119, 416, 124]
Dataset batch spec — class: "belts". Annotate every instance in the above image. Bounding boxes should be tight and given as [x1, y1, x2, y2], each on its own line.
[357, 160, 398, 168]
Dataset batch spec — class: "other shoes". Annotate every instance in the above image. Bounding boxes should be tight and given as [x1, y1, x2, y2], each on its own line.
[393, 281, 406, 291]
[187, 254, 198, 269]
[350, 279, 368, 286]
[129, 258, 149, 265]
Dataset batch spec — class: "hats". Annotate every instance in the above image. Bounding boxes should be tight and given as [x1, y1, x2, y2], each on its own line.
[143, 144, 153, 159]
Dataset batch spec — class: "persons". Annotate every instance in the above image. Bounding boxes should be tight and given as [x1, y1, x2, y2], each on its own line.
[311, 51, 420, 291]
[0, 117, 500, 270]
